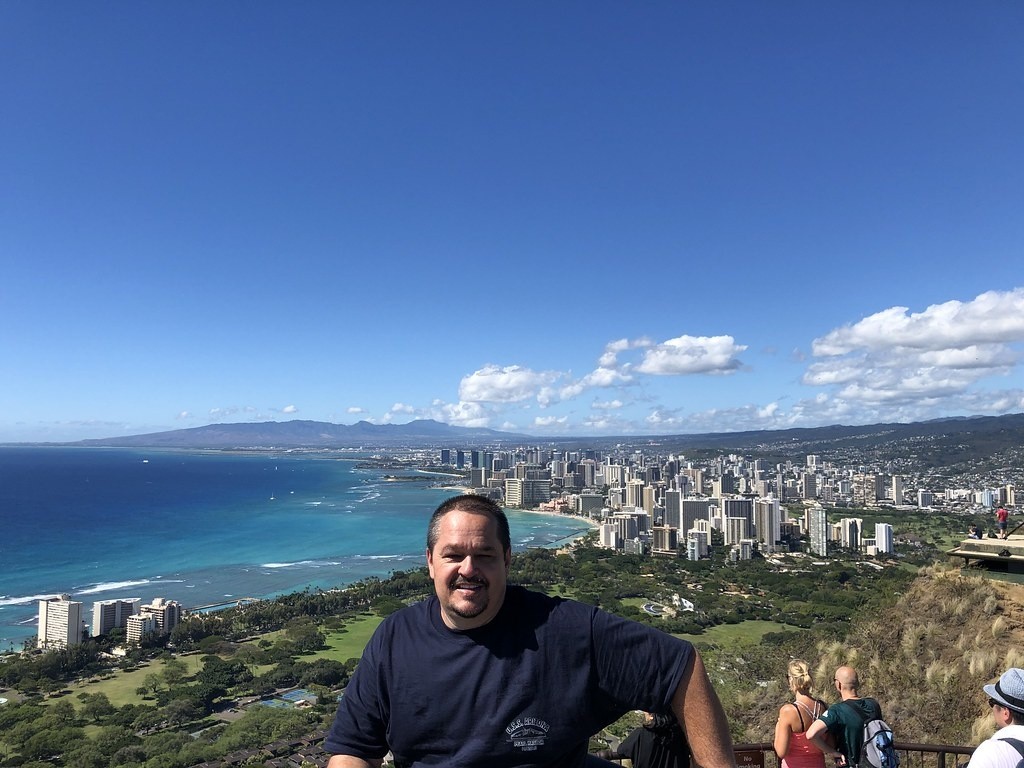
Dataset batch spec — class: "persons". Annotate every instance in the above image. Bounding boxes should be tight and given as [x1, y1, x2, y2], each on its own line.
[806, 667, 883, 768]
[967, 523, 982, 539]
[323, 494, 737, 768]
[964, 668, 1024, 768]
[995, 506, 1008, 538]
[615, 709, 692, 768]
[773, 659, 831, 768]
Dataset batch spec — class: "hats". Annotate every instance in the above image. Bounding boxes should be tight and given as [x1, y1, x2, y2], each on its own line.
[983, 667, 1024, 713]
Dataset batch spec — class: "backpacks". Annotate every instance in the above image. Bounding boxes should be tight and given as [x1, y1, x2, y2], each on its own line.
[842, 699, 901, 768]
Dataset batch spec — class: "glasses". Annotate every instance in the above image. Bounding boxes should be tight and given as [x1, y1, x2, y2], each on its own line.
[989, 699, 1005, 708]
[786, 674, 791, 679]
[833, 678, 844, 685]
